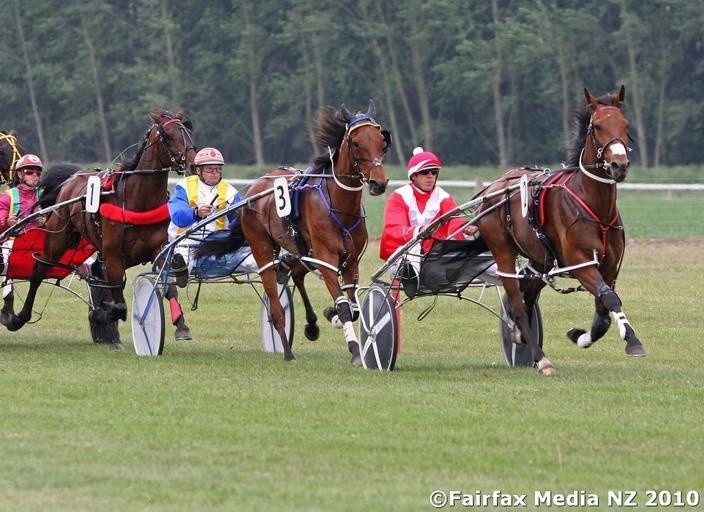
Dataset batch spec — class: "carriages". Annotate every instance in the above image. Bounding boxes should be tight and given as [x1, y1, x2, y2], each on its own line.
[0, 84, 643, 376]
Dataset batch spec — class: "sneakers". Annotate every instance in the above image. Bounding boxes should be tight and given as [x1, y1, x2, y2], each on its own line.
[402, 265, 417, 299]
[276, 252, 299, 284]
[172, 254, 188, 288]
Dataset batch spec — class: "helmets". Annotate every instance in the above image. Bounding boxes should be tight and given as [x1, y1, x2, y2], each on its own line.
[194, 148, 225, 165]
[408, 147, 442, 181]
[16, 154, 43, 169]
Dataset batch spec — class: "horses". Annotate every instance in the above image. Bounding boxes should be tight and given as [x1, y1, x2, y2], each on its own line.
[427, 84, 648, 378]
[0, 106, 198, 351]
[0, 129, 24, 326]
[190, 99, 390, 368]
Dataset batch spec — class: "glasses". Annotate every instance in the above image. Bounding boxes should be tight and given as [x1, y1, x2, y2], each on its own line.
[418, 168, 439, 175]
[204, 167, 222, 172]
[23, 169, 41, 177]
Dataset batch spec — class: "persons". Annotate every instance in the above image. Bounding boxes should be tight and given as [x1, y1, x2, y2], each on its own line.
[0, 153, 102, 304]
[164, 147, 290, 288]
[378, 146, 533, 302]
